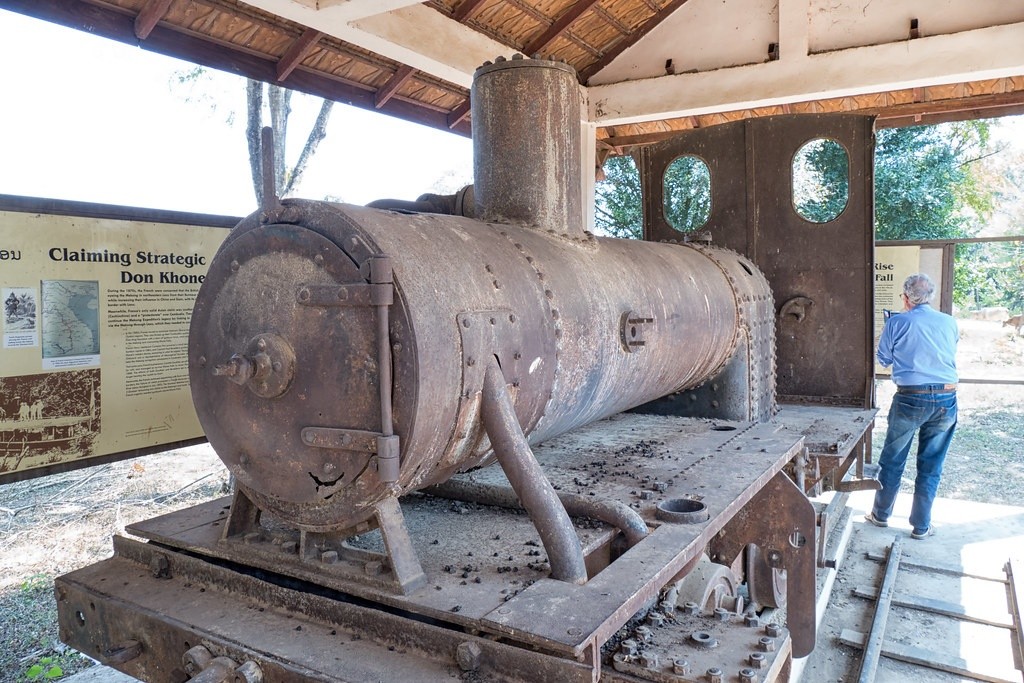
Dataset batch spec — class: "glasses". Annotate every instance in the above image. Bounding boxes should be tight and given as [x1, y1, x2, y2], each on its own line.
[899, 292, 908, 300]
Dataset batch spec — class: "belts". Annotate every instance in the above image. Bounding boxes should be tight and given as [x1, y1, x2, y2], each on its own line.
[897, 388, 955, 393]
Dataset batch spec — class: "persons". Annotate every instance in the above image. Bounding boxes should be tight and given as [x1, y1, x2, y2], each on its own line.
[19, 400, 44, 422]
[0, 407, 6, 423]
[864, 273, 959, 540]
[5, 293, 19, 321]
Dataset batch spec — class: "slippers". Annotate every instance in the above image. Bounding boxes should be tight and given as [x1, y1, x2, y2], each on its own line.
[911, 521, 937, 540]
[865, 508, 888, 527]
